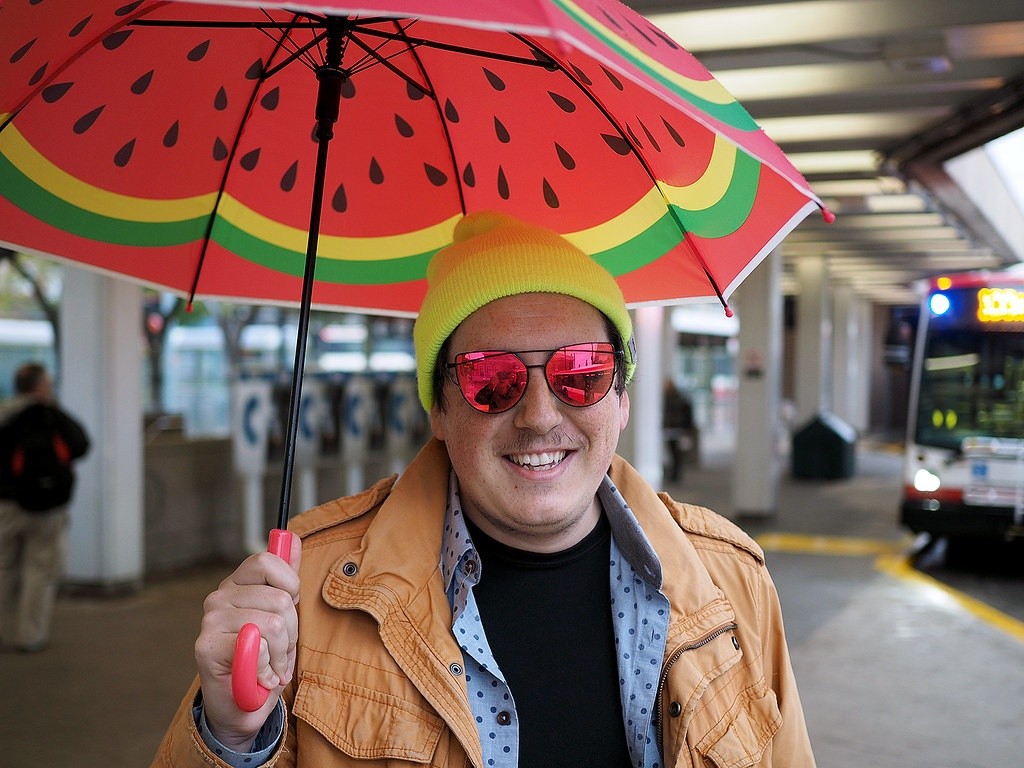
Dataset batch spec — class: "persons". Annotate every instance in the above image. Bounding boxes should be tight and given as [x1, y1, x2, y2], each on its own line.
[154, 208, 813, 768]
[0, 362, 95, 659]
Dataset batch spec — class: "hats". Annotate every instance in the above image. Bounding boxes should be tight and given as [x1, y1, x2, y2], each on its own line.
[413, 211, 637, 417]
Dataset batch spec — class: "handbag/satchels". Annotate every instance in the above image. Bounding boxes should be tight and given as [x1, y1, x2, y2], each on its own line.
[15, 414, 73, 514]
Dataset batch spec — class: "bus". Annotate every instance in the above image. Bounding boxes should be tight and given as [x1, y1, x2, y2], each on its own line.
[1, 311, 418, 440]
[894, 269, 1023, 581]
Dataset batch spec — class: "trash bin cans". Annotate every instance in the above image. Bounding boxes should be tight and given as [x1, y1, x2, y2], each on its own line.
[791, 415, 858, 479]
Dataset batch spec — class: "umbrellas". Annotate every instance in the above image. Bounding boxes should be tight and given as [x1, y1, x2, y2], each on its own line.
[0, 0, 838, 708]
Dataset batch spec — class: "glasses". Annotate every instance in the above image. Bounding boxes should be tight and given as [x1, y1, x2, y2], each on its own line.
[437, 342, 625, 415]
